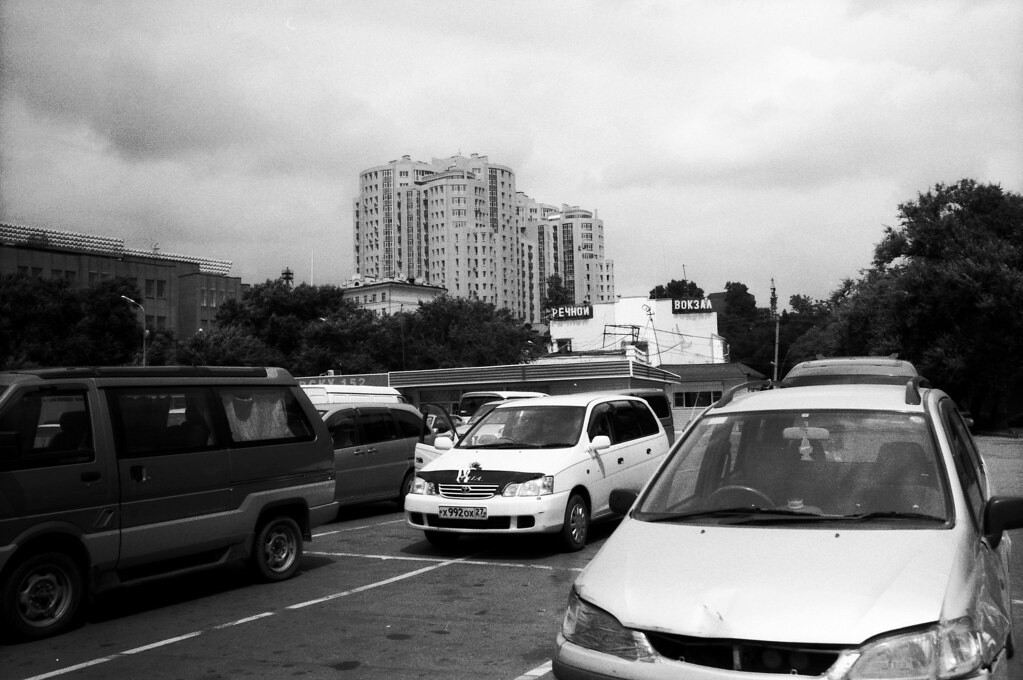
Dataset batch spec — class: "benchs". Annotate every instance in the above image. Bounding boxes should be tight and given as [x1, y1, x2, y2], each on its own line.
[798, 460, 875, 502]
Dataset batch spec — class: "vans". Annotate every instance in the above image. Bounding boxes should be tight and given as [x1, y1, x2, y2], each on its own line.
[0, 364, 337, 639]
[297, 383, 405, 410]
[457, 390, 551, 422]
[313, 404, 435, 505]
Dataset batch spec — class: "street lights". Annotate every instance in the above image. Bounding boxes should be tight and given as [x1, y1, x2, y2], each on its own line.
[121, 296, 145, 366]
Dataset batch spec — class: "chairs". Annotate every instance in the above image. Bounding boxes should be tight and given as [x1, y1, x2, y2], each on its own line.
[45, 410, 89, 459]
[721, 443, 806, 505]
[162, 407, 210, 448]
[849, 441, 946, 517]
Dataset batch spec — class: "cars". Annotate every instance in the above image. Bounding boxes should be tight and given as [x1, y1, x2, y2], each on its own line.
[403, 391, 670, 552]
[550, 359, 1023, 680]
